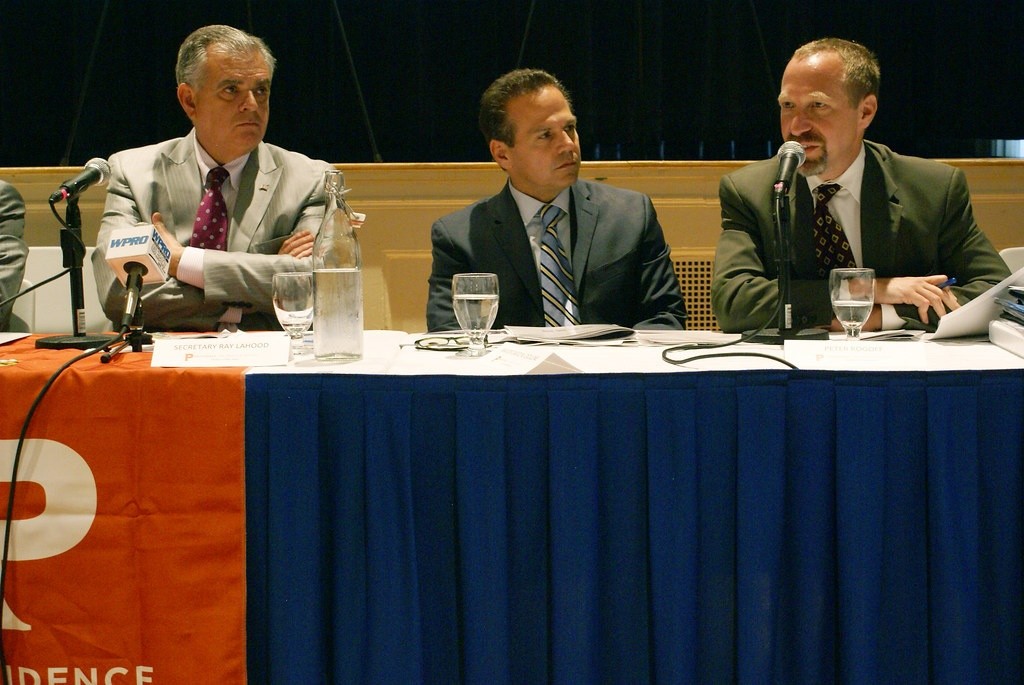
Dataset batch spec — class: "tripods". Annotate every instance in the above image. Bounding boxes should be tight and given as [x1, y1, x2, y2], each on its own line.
[100, 296, 157, 363]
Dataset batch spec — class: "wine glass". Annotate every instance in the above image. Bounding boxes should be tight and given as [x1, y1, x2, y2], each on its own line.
[452, 272, 499, 359]
[273, 272, 316, 355]
[829, 268, 876, 342]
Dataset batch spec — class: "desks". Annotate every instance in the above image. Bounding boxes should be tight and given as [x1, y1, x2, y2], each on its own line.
[0, 327, 1023, 685]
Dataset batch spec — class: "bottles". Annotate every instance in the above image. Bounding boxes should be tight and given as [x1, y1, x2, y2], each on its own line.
[312, 169, 364, 363]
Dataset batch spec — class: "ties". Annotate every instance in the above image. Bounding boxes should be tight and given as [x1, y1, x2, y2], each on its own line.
[533, 204, 582, 328]
[812, 183, 858, 281]
[190, 166, 230, 251]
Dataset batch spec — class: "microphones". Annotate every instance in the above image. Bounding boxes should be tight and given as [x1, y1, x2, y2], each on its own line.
[105, 222, 171, 331]
[774, 140, 806, 200]
[49, 157, 111, 205]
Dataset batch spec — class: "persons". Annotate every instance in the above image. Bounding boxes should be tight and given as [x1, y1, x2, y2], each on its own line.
[711, 38, 1011, 334]
[0, 179, 29, 333]
[426, 68, 687, 334]
[91, 25, 341, 332]
